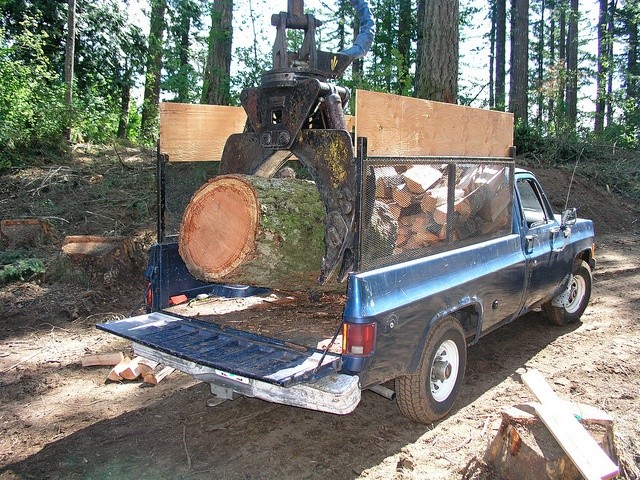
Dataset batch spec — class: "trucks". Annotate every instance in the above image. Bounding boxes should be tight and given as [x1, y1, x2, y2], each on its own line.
[92, 138, 596, 425]
